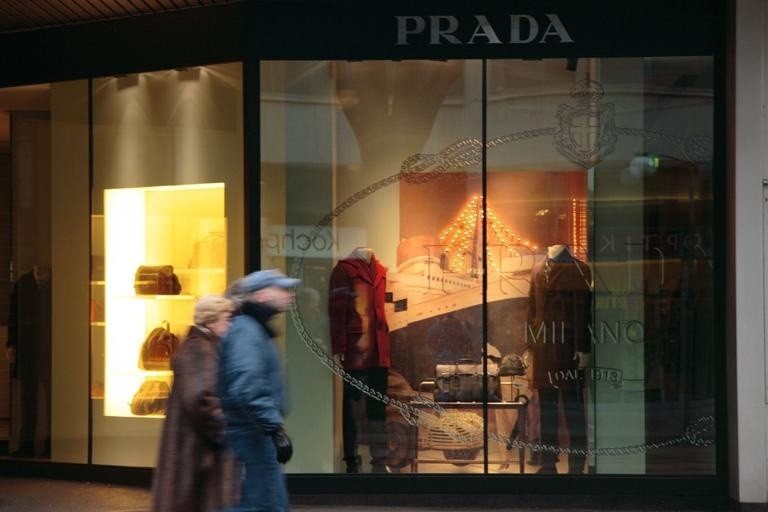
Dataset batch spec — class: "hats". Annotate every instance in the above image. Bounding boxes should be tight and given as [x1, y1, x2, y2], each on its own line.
[500, 353, 524, 376]
[239, 267, 302, 292]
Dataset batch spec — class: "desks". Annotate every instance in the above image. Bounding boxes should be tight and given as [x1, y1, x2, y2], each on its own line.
[405, 400, 529, 474]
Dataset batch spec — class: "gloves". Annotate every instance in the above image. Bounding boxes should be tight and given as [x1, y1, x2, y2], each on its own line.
[273, 428, 293, 463]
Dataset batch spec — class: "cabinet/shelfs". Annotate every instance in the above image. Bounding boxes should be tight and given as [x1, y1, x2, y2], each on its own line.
[101, 182, 230, 421]
[88, 213, 106, 401]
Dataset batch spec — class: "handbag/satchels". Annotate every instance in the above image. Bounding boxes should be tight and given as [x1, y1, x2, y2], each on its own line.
[134, 266, 181, 295]
[433, 359, 501, 402]
[129, 380, 171, 415]
[139, 320, 181, 371]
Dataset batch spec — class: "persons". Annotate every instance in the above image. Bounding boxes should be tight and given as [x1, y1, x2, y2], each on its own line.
[518, 241, 592, 474]
[5, 256, 53, 461]
[328, 244, 393, 476]
[215, 269, 305, 511]
[220, 277, 247, 313]
[149, 297, 245, 511]
